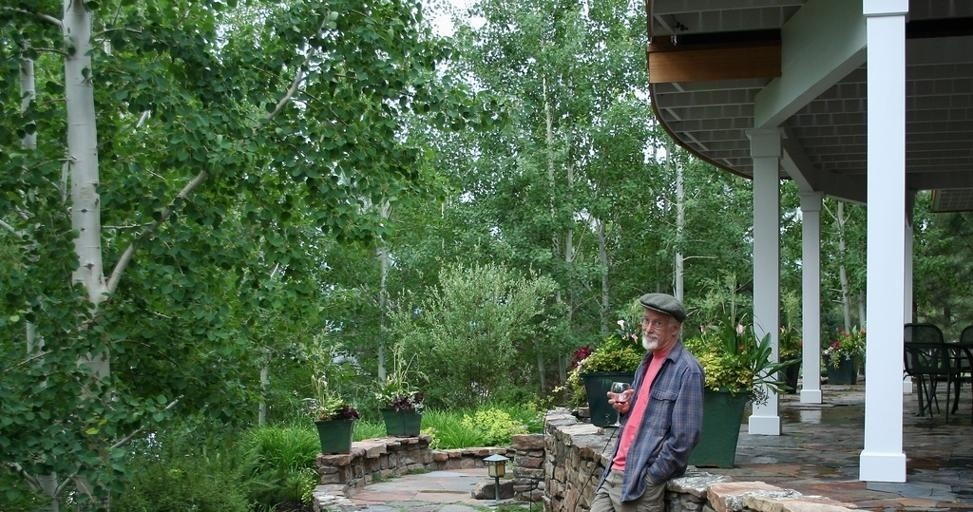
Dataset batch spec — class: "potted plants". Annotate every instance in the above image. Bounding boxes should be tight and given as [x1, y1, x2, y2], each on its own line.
[376, 347, 430, 439]
[779, 324, 802, 393]
[576, 336, 642, 428]
[304, 375, 360, 454]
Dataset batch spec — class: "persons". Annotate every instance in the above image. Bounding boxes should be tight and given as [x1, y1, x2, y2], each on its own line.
[588, 290, 704, 511]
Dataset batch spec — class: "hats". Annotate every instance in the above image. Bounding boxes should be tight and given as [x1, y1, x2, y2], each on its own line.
[638, 293, 687, 321]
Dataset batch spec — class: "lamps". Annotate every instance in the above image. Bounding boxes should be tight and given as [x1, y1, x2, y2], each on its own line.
[484, 452, 511, 499]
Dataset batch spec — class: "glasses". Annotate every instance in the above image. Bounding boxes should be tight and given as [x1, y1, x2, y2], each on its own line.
[641, 317, 667, 327]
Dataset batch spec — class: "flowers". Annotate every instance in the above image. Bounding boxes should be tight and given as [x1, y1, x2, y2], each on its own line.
[685, 312, 803, 407]
[822, 323, 866, 370]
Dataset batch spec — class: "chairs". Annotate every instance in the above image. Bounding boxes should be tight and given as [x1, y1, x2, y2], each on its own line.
[904, 324, 973, 423]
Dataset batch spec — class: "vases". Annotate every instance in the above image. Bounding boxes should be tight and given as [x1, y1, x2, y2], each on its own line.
[826, 356, 856, 384]
[687, 387, 752, 469]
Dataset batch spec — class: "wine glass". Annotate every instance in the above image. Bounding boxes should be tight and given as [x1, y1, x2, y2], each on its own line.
[610, 383, 631, 428]
